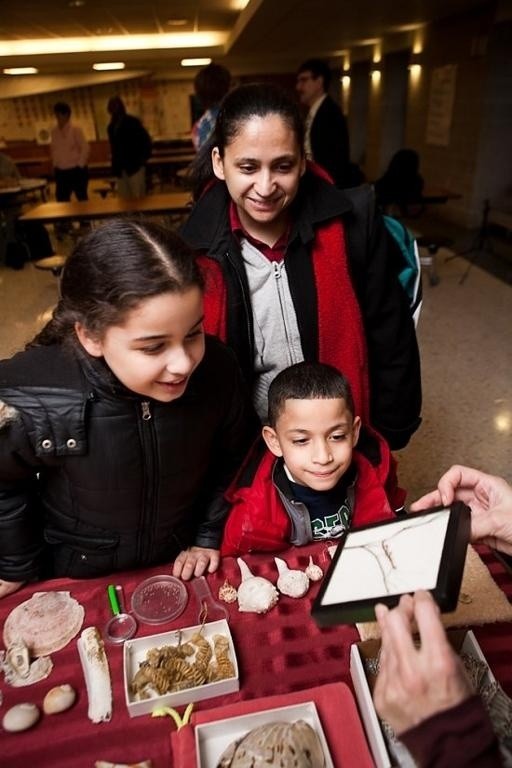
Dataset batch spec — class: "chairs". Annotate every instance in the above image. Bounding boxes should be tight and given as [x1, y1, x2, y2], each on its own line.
[367, 143, 438, 258]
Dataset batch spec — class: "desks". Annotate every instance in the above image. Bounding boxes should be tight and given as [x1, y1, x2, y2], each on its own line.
[0, 515, 511, 767]
[1, 142, 208, 303]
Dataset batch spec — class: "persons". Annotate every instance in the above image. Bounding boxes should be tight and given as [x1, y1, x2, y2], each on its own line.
[188, 62, 231, 154]
[173, 82, 424, 451]
[51, 102, 90, 226]
[107, 95, 150, 195]
[374, 150, 424, 201]
[0, 220, 263, 596]
[373, 464, 512, 768]
[220, 362, 408, 554]
[296, 57, 350, 184]
